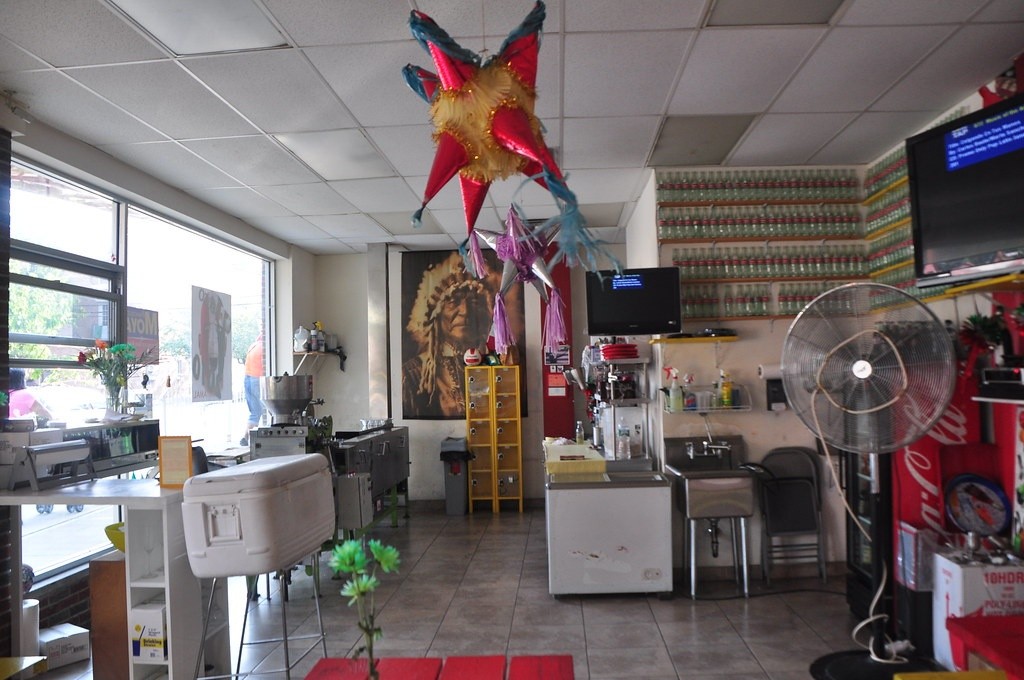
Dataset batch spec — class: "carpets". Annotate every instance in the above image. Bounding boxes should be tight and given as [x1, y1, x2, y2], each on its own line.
[303, 654, 574, 680]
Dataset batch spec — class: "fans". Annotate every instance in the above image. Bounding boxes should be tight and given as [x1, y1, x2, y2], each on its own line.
[782, 283, 958, 680]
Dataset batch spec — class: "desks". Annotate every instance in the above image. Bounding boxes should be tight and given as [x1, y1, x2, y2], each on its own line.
[0, 478, 232, 680]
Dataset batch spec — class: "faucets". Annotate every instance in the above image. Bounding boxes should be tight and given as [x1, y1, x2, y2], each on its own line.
[702, 441, 731, 455]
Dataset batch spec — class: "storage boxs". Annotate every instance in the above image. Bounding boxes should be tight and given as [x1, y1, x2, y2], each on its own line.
[129, 603, 168, 662]
[39, 623, 90, 671]
[181, 453, 337, 578]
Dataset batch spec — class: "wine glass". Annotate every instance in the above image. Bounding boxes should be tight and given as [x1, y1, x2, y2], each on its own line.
[965, 532, 981, 565]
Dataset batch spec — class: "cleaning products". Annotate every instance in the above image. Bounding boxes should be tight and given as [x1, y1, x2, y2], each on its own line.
[663, 365, 732, 412]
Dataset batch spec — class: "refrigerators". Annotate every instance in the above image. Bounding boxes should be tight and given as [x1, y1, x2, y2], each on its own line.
[544, 471, 673, 599]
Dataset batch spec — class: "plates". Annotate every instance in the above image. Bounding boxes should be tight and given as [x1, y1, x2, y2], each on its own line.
[62, 428, 136, 460]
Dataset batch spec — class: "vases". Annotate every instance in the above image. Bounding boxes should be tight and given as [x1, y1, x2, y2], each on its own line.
[105, 384, 122, 412]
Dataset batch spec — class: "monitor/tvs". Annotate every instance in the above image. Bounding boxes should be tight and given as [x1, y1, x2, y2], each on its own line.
[586, 267, 681, 336]
[905, 91, 1024, 289]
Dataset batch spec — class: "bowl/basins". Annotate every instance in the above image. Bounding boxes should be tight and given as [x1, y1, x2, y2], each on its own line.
[104, 523, 125, 553]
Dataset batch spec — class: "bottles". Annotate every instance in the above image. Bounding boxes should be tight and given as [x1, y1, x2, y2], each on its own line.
[590, 336, 628, 362]
[852, 454, 871, 566]
[617, 416, 631, 460]
[655, 105, 953, 319]
[576, 420, 584, 445]
[593, 418, 602, 450]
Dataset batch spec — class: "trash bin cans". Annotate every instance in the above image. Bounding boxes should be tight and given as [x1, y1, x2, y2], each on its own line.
[441, 436, 468, 517]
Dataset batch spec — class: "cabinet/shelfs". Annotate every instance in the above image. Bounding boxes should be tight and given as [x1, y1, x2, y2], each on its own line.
[592, 357, 654, 472]
[841, 354, 980, 648]
[465, 365, 523, 513]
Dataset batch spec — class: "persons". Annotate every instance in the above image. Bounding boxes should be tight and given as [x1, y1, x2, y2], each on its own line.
[240, 335, 268, 447]
[9, 368, 51, 428]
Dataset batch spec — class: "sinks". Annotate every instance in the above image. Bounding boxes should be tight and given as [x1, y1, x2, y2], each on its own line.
[664, 464, 764, 519]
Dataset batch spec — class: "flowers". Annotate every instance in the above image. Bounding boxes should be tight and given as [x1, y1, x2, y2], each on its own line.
[78, 337, 162, 412]
[324, 536, 402, 680]
